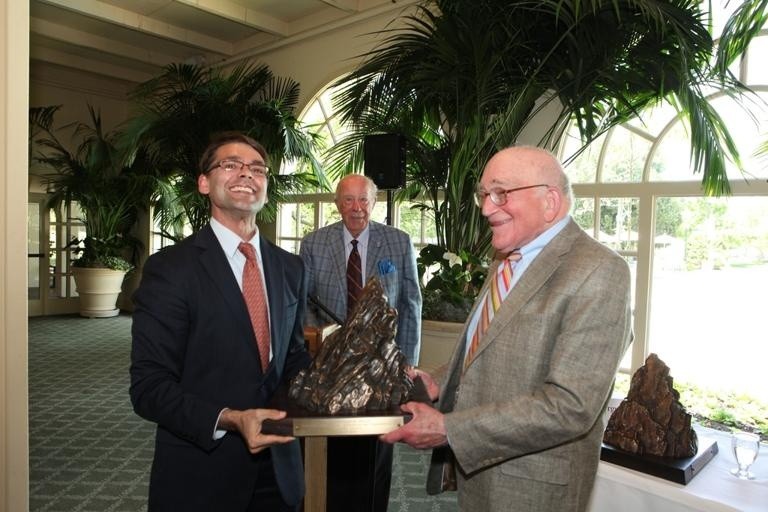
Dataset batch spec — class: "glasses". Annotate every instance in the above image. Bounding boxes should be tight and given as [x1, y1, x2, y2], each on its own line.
[472, 184, 549, 210]
[204, 158, 271, 178]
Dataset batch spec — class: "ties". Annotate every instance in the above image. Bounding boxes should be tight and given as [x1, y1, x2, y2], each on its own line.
[238, 242, 270, 375]
[464, 248, 522, 372]
[346, 240, 362, 319]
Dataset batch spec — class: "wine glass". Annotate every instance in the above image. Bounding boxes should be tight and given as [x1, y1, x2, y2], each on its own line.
[729, 432, 759, 480]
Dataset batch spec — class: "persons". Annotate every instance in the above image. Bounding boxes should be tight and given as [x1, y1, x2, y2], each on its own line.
[127, 127, 319, 510]
[302, 174, 424, 512]
[378, 141, 637, 510]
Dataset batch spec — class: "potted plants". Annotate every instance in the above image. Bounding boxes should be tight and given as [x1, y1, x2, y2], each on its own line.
[331, 8, 763, 391]
[26, 72, 187, 320]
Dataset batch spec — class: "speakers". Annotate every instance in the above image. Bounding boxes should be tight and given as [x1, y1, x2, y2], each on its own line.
[363, 132, 407, 191]
